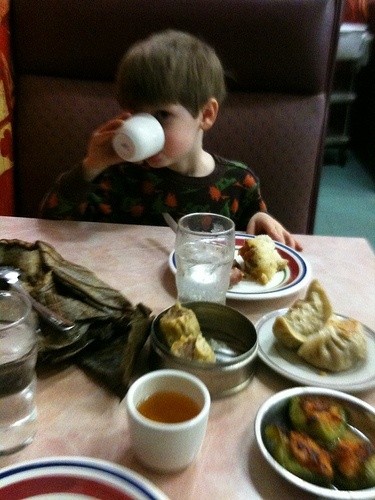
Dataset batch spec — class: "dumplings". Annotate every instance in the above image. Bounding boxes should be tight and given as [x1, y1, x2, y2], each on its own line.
[298, 318, 367, 373]
[270, 277, 334, 348]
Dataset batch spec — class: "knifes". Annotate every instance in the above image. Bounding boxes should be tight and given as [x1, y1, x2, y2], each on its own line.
[163, 212, 241, 269]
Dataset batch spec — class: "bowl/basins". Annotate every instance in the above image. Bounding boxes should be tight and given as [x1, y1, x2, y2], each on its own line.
[255, 387, 375, 500]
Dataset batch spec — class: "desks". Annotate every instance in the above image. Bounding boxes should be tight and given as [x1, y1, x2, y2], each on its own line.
[0, 213, 375, 500]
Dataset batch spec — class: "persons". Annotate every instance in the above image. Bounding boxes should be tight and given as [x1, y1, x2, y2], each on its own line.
[39, 30, 304, 252]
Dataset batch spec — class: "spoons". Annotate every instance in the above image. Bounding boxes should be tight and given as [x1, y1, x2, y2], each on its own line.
[0, 266, 76, 331]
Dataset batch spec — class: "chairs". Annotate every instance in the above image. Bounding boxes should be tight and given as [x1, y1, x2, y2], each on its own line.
[8, 0, 344, 234]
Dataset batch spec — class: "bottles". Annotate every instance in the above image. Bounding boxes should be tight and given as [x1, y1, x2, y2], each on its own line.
[0, 291, 39, 456]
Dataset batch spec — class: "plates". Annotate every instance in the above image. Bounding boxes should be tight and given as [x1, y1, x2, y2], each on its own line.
[0, 457, 170, 500]
[169, 234, 311, 301]
[255, 308, 375, 394]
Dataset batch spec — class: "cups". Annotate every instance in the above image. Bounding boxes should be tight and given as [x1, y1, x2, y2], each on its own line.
[175, 212, 235, 305]
[112, 112, 165, 162]
[127, 369, 210, 475]
